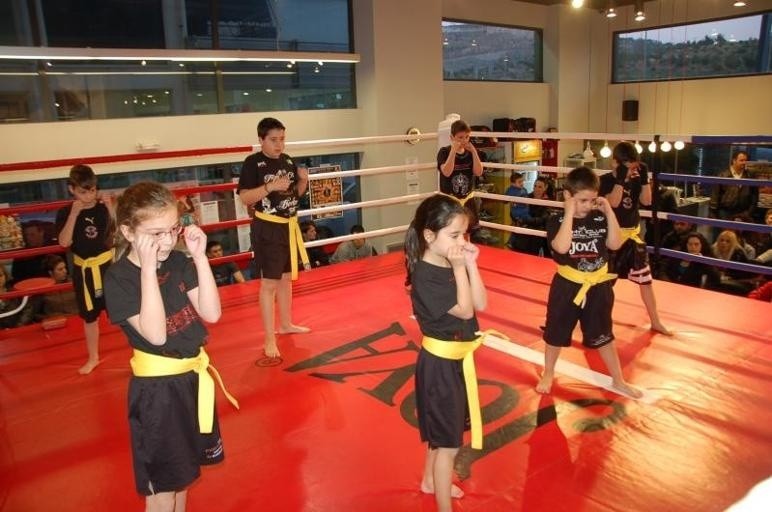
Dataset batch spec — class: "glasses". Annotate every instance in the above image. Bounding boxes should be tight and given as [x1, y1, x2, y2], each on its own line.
[137, 223, 183, 240]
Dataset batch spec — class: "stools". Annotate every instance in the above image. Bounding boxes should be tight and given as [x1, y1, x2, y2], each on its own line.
[0, 276, 57, 319]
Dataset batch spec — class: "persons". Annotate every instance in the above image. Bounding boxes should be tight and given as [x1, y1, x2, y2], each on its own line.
[205, 241, 245, 287]
[299, 221, 330, 268]
[595, 141, 675, 336]
[398, 194, 489, 512]
[237, 117, 311, 359]
[328, 225, 371, 263]
[535, 166, 643, 402]
[0, 220, 79, 328]
[438, 121, 483, 242]
[102, 180, 224, 512]
[56, 163, 117, 377]
[503, 175, 772, 301]
[710, 150, 759, 241]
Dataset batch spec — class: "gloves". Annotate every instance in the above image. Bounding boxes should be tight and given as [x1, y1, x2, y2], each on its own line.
[614, 164, 629, 188]
[637, 161, 651, 186]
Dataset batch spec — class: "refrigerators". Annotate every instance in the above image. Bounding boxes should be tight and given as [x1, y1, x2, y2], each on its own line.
[468, 140, 543, 252]
